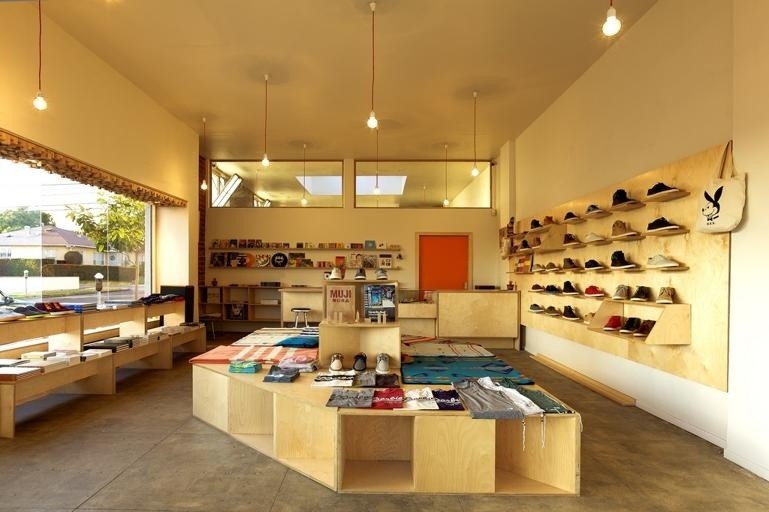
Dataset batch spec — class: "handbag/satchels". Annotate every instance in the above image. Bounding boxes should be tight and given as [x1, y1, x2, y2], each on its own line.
[695, 175, 746, 234]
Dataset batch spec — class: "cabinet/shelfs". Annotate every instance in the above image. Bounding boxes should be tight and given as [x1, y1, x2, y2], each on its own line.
[278, 286, 325, 328]
[505, 189, 691, 346]
[317, 280, 402, 369]
[199, 285, 282, 322]
[208, 247, 401, 270]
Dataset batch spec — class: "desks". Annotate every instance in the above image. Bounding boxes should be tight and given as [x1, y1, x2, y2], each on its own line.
[0, 322, 206, 439]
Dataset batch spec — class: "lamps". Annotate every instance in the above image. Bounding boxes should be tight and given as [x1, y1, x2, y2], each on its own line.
[443, 144, 449, 205]
[471, 90, 480, 176]
[301, 144, 307, 206]
[603, 2, 621, 37]
[375, 127, 379, 193]
[367, 1, 378, 128]
[262, 74, 270, 165]
[34, 0, 48, 110]
[200, 118, 208, 189]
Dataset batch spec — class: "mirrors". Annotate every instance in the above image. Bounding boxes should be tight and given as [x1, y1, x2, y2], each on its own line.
[353, 160, 491, 209]
[209, 160, 344, 208]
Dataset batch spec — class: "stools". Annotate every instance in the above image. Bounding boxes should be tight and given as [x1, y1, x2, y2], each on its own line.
[199, 316, 216, 344]
[290, 307, 312, 329]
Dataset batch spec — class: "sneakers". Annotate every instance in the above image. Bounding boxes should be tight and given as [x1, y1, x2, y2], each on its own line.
[377, 268, 388, 279]
[329, 352, 343, 374]
[376, 354, 391, 374]
[520, 183, 680, 336]
[355, 267, 365, 279]
[353, 351, 367, 372]
[331, 266, 341, 279]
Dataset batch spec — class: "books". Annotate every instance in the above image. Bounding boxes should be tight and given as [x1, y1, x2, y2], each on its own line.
[326, 285, 356, 322]
[0, 322, 205, 381]
[364, 282, 396, 322]
